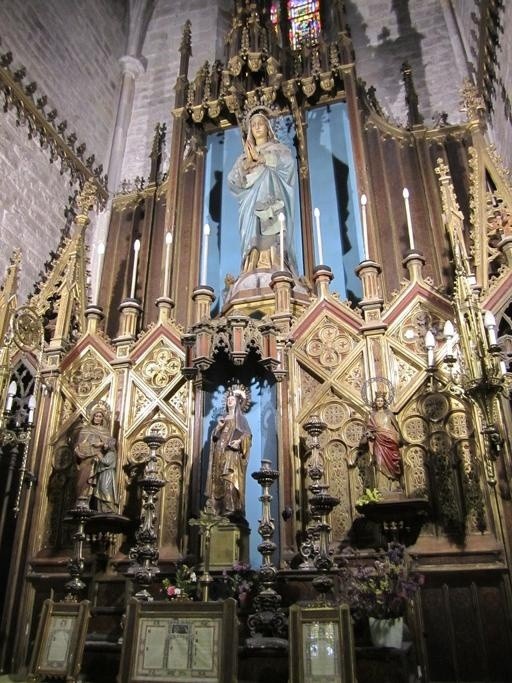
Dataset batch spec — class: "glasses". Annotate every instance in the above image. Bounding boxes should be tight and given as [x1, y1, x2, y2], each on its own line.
[426, 229, 506, 452]
[0, 327, 37, 456]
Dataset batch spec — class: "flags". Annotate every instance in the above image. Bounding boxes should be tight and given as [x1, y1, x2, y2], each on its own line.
[366, 617, 404, 648]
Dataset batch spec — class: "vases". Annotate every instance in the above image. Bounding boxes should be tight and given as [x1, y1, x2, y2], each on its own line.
[343, 544, 425, 627]
[161, 565, 196, 599]
[221, 562, 253, 604]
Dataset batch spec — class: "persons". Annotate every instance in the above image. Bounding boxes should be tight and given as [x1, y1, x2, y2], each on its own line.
[74, 407, 117, 514]
[227, 114, 295, 274]
[203, 395, 252, 516]
[365, 396, 411, 494]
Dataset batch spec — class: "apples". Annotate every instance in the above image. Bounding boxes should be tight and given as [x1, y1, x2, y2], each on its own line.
[92, 242, 105, 305]
[314, 207, 325, 264]
[130, 238, 141, 299]
[278, 213, 285, 272]
[162, 232, 173, 296]
[200, 223, 210, 286]
[360, 193, 371, 259]
[402, 187, 416, 250]
